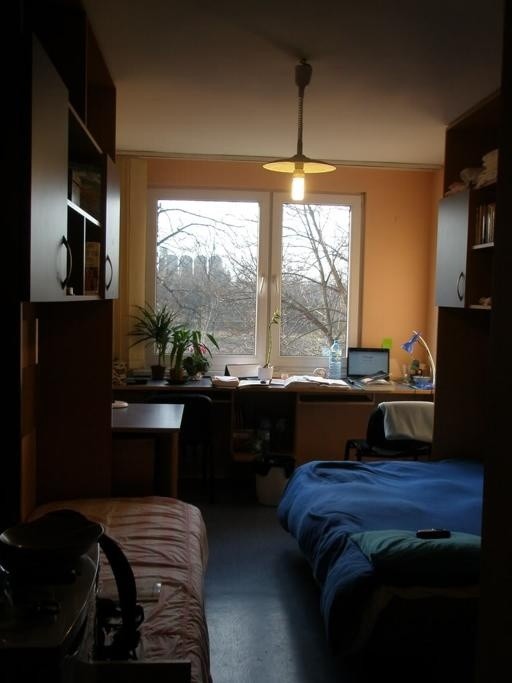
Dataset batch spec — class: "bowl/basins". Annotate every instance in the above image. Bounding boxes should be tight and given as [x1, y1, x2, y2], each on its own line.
[412, 375, 431, 384]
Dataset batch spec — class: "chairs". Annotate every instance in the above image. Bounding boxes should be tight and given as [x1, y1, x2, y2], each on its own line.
[228, 384, 297, 499]
[153, 393, 215, 505]
[343, 400, 433, 460]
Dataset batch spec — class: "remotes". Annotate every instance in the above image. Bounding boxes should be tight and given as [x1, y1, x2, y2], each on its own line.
[260, 378, 266, 383]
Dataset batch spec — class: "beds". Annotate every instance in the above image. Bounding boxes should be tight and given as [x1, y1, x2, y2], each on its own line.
[276, 460, 484, 641]
[25, 493, 210, 681]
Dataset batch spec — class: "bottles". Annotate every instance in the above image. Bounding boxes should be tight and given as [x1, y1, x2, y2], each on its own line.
[329, 339, 342, 379]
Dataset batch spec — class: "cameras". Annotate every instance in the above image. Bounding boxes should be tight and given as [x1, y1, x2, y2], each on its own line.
[416, 528, 450, 538]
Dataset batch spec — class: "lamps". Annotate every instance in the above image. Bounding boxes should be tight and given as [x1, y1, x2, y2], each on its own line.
[399, 329, 436, 389]
[261, 60, 339, 203]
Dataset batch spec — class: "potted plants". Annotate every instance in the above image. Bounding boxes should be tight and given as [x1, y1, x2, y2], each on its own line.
[169, 326, 221, 382]
[257, 305, 282, 382]
[122, 302, 179, 380]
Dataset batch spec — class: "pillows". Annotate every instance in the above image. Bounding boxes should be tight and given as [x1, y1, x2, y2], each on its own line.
[348, 526, 480, 572]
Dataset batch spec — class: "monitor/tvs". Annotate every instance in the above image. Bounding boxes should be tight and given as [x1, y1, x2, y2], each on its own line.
[347, 347, 389, 384]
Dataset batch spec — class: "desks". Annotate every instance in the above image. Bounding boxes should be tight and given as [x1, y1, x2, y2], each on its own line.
[111, 403, 185, 497]
[112, 375, 434, 398]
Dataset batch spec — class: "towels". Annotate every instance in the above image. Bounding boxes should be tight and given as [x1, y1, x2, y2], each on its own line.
[377, 400, 435, 444]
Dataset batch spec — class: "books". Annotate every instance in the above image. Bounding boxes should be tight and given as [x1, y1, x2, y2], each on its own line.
[211, 369, 391, 389]
[473, 201, 496, 246]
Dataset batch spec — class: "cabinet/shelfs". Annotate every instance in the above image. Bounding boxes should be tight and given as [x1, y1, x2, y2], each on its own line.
[29, 42, 122, 302]
[435, 87, 499, 310]
